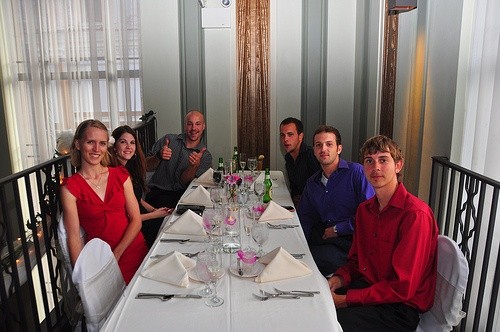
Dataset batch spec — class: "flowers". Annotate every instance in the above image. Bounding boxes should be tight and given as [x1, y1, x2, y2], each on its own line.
[253, 205, 266, 212]
[238, 250, 257, 263]
[224, 215, 236, 225]
[225, 174, 252, 186]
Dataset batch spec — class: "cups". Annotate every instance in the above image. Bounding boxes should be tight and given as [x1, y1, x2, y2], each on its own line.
[204, 209, 221, 232]
[241, 248, 259, 274]
[249, 194, 264, 217]
[225, 159, 236, 173]
[222, 204, 242, 253]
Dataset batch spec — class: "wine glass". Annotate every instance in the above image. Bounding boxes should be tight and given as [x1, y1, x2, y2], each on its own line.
[238, 153, 269, 256]
[203, 172, 223, 253]
[197, 249, 225, 307]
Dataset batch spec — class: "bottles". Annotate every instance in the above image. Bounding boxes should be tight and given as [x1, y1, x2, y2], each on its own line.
[218, 157, 224, 174]
[263, 168, 273, 203]
[232, 147, 239, 173]
[272, 177, 279, 188]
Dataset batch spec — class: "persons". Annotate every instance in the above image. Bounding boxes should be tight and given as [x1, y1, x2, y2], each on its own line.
[297, 125, 376, 277]
[279, 117, 321, 208]
[58, 119, 149, 286]
[143, 110, 212, 208]
[107, 125, 173, 249]
[325, 135, 438, 332]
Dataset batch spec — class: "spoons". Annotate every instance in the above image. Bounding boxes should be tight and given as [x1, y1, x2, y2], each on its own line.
[161, 238, 191, 242]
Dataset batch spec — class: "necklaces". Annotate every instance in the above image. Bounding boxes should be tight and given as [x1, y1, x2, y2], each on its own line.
[78, 167, 103, 189]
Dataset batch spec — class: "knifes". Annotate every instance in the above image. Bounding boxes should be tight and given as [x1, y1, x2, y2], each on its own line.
[138, 293, 202, 298]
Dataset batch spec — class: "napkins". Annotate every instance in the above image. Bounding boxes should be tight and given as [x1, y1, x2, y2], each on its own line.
[180, 184, 213, 207]
[193, 168, 217, 186]
[258, 200, 295, 222]
[163, 209, 209, 236]
[249, 172, 280, 191]
[253, 245, 312, 283]
[140, 250, 196, 288]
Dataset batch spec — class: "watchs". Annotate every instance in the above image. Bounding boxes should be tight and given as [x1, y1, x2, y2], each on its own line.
[333, 224, 337, 232]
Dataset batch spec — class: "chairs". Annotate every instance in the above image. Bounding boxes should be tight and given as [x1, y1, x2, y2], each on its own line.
[413, 235, 469, 332]
[57, 211, 126, 332]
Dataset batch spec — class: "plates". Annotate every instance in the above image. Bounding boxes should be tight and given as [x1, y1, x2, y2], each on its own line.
[206, 227, 223, 235]
[245, 206, 266, 219]
[230, 262, 265, 278]
[189, 264, 226, 281]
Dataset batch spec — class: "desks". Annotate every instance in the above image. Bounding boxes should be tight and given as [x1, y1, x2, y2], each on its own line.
[100, 171, 343, 332]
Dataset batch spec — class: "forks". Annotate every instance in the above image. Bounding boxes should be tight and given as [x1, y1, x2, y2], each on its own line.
[253, 288, 320, 301]
[150, 251, 199, 258]
[267, 222, 300, 227]
[135, 295, 175, 300]
